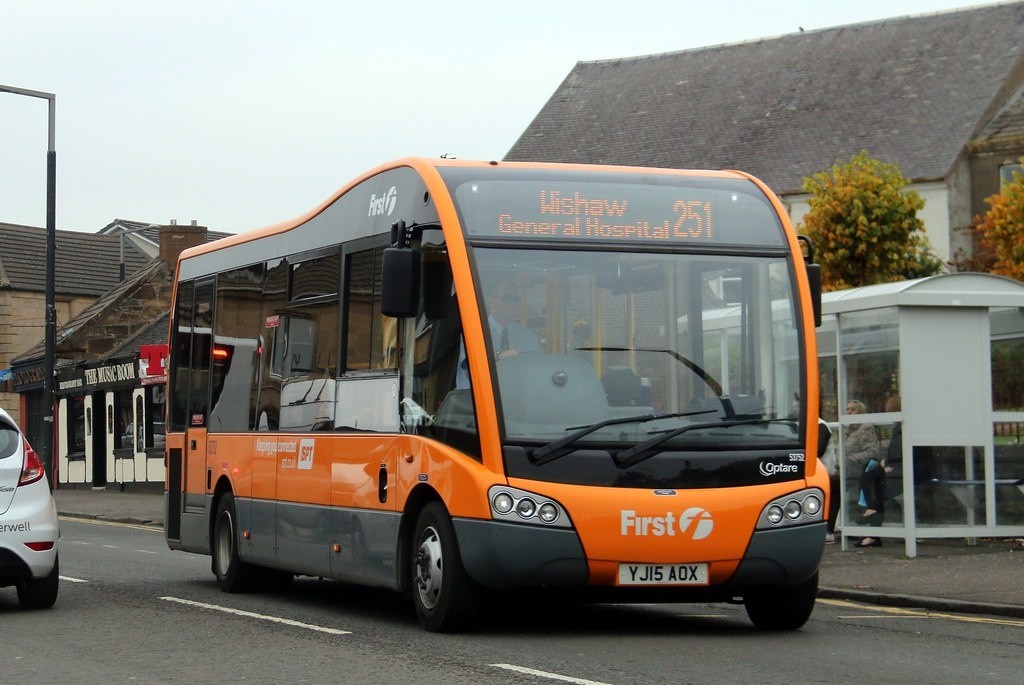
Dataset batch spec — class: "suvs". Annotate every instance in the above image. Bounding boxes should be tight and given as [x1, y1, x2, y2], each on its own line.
[0, 405, 62, 610]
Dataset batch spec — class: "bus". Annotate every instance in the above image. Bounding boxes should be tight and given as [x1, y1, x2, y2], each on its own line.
[160, 152, 832, 634]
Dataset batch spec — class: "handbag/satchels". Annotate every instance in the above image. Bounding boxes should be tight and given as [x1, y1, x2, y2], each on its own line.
[858, 459, 879, 507]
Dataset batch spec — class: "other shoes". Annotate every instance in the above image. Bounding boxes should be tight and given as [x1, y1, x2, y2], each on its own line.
[825, 533, 835, 544]
[854, 540, 882, 547]
[855, 510, 881, 524]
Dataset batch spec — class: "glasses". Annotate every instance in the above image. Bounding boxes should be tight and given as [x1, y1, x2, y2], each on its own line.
[492, 294, 521, 302]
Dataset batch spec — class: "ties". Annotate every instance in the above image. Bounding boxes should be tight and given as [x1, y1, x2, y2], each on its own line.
[501, 328, 509, 351]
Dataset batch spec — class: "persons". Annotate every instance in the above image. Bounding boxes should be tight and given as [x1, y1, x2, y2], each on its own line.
[853, 394, 934, 547]
[825, 399, 879, 545]
[454, 280, 546, 391]
[787, 394, 836, 483]
